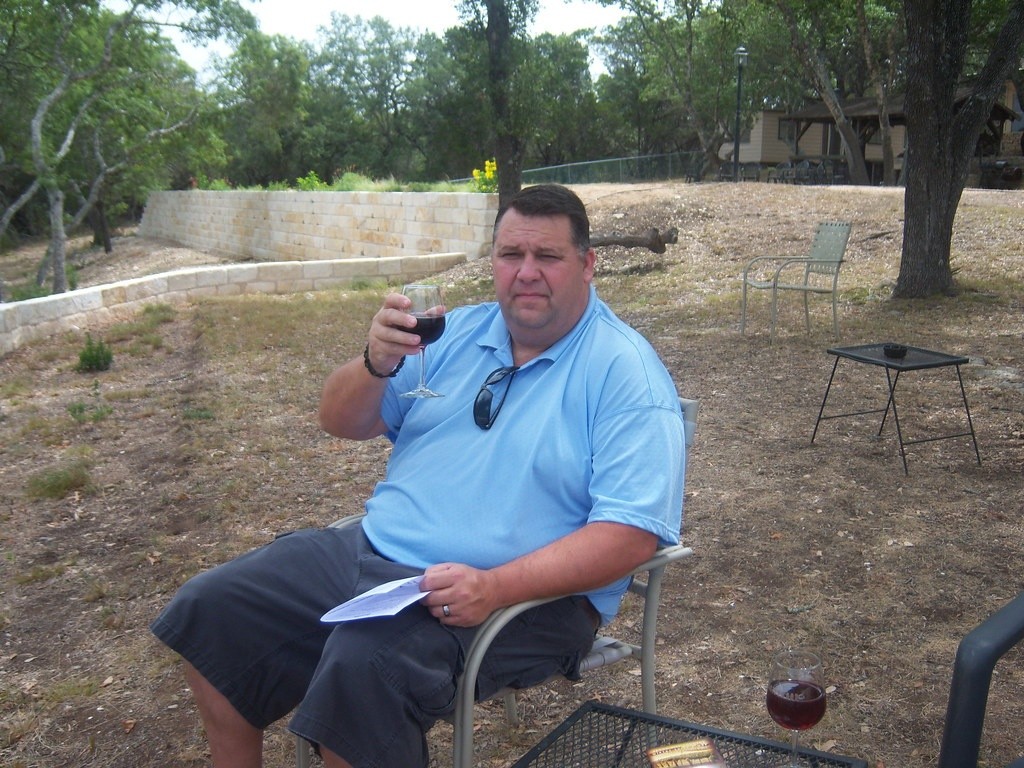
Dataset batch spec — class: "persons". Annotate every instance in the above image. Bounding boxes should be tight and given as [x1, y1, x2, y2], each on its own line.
[152, 182, 686, 768]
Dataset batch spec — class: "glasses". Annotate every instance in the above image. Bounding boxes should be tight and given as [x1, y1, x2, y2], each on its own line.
[472, 365, 520, 430]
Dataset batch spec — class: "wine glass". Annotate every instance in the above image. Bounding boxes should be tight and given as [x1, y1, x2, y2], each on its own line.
[766, 652, 827, 767]
[397, 285, 443, 399]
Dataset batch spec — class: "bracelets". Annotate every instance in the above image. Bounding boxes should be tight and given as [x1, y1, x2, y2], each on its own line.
[364, 344, 405, 378]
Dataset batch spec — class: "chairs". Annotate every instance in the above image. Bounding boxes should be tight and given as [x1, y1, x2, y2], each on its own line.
[939, 589, 1024, 768]
[739, 221, 854, 341]
[296, 512, 695, 768]
[684, 158, 846, 186]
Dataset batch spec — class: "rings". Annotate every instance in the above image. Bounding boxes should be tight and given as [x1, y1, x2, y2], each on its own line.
[443, 606, 450, 617]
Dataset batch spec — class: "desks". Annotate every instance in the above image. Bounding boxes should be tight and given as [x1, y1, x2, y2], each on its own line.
[515, 700, 868, 768]
[810, 342, 981, 476]
